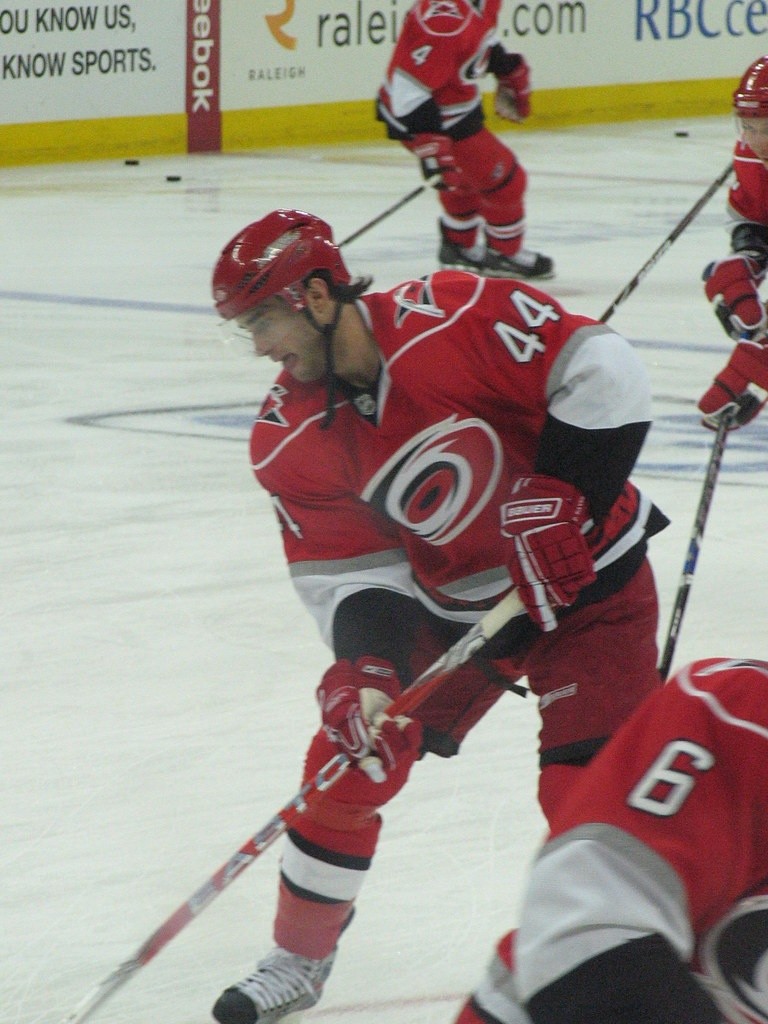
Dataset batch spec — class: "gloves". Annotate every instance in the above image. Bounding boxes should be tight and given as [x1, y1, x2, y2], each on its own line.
[418, 134, 461, 193]
[697, 337, 768, 431]
[493, 54, 530, 125]
[497, 475, 596, 634]
[317, 655, 426, 785]
[703, 255, 767, 337]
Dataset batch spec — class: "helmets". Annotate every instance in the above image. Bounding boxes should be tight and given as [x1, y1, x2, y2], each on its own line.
[733, 54, 768, 117]
[211, 210, 350, 322]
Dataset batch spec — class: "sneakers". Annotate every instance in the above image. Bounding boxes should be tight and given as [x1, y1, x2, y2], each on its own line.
[438, 241, 489, 271]
[213, 942, 337, 1024]
[483, 245, 554, 280]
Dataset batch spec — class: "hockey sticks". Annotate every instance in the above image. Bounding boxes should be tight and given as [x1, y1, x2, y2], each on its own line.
[64, 593, 526, 1024]
[596, 156, 736, 324]
[339, 180, 427, 246]
[660, 411, 732, 678]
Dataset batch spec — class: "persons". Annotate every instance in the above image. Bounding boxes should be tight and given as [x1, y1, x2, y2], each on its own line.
[209, 208, 671, 1024]
[455, 655, 768, 1024]
[696, 52, 768, 433]
[373, 0, 557, 280]
